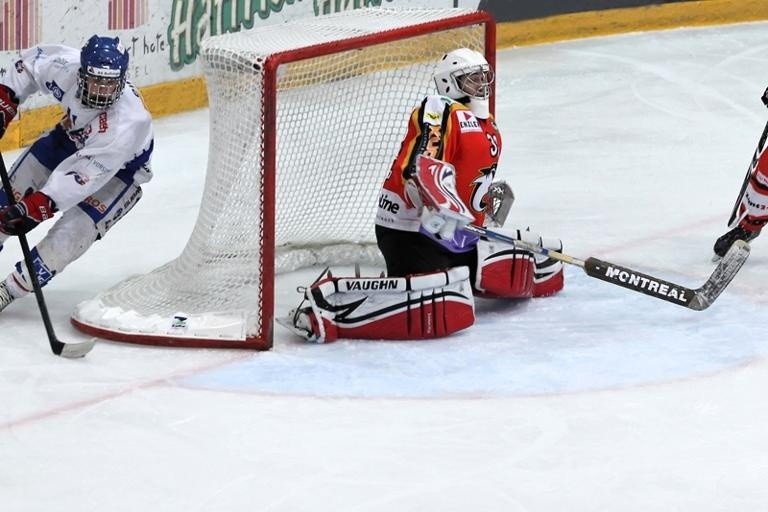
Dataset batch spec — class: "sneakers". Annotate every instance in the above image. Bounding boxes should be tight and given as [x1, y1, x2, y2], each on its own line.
[714, 224, 761, 259]
[0, 271, 29, 312]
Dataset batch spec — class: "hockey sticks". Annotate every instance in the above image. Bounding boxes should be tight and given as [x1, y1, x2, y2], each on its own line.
[0, 156, 97, 359]
[464, 222, 750, 311]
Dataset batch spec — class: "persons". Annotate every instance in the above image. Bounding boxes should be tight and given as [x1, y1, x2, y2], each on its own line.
[289, 47, 565, 343]
[0, 34, 153, 314]
[715, 85, 768, 257]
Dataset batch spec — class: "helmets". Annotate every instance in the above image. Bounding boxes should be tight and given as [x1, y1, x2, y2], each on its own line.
[432, 48, 496, 120]
[76, 34, 130, 110]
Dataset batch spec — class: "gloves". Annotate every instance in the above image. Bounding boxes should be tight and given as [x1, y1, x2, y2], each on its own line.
[0, 82, 20, 138]
[0, 187, 54, 236]
